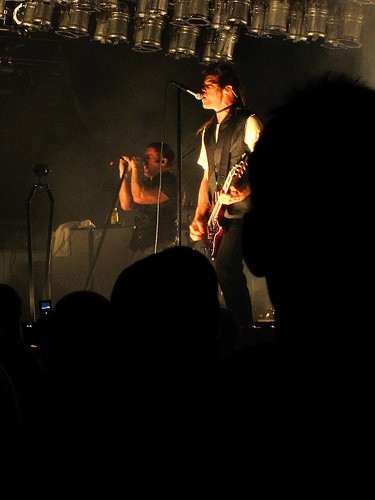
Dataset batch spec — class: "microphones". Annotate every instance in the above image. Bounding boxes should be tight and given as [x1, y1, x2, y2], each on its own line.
[169, 81, 204, 100]
[123, 159, 148, 166]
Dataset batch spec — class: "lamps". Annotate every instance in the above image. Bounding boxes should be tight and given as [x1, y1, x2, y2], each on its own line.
[0, 0, 375, 61]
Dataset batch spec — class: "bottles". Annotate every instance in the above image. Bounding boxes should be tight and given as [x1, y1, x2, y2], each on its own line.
[111, 208, 120, 223]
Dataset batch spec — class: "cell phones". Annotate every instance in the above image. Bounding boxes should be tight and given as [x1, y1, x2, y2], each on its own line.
[39, 300, 52, 317]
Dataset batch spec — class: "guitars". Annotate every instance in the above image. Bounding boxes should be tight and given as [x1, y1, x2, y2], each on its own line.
[201, 149, 249, 258]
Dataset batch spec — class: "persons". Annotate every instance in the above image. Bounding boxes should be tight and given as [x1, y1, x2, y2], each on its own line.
[0, 245, 374, 500]
[188, 63, 265, 330]
[237, 69, 374, 500]
[118, 141, 185, 265]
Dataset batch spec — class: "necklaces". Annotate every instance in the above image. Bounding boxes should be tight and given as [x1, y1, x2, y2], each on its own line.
[216, 103, 235, 114]
